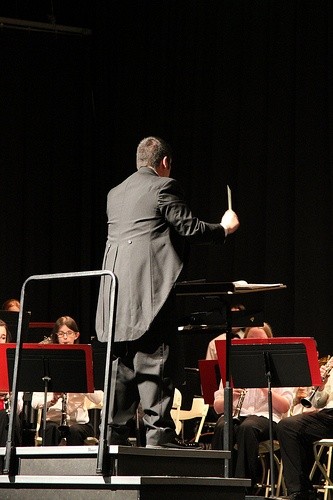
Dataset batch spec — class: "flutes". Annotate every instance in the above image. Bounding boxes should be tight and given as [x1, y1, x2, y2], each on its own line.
[301, 366, 333, 408]
[58, 343, 69, 431]
[4, 394, 12, 416]
[233, 387, 248, 422]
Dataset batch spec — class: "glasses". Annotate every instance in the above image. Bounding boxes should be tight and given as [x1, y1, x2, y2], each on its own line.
[57, 331, 74, 337]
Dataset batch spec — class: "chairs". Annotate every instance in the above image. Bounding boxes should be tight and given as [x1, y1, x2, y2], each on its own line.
[32, 367, 333, 500]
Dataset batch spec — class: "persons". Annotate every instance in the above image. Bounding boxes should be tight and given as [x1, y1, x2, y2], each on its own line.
[93, 137, 241, 447]
[280, 337, 333, 500]
[212, 322, 296, 491]
[0, 298, 105, 446]
[204, 303, 248, 360]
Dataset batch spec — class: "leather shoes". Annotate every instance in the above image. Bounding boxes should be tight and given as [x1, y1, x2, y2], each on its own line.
[146, 435, 202, 450]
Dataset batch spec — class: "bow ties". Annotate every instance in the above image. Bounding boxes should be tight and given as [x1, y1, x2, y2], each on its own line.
[231, 334, 240, 339]
